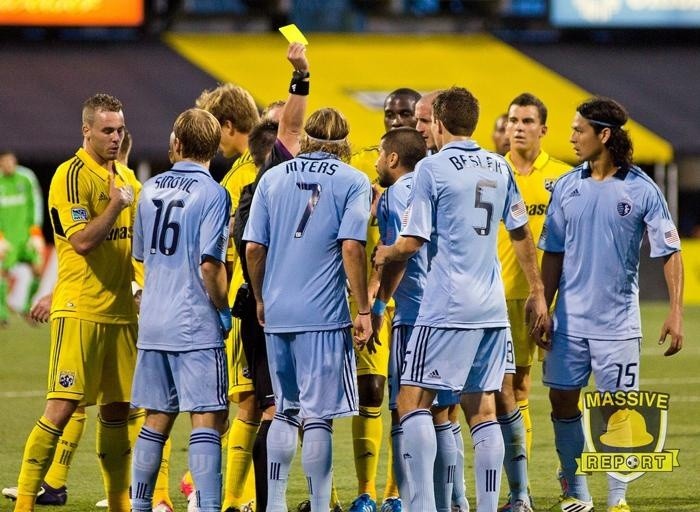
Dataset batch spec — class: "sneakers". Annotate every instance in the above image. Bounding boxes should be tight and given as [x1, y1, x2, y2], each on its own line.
[548, 492, 594, 512]
[607, 502, 630, 512]
[498, 494, 534, 511]
[180, 469, 199, 512]
[2, 481, 66, 506]
[381, 497, 402, 512]
[298, 500, 343, 511]
[556, 462, 569, 498]
[153, 501, 172, 512]
[349, 493, 376, 512]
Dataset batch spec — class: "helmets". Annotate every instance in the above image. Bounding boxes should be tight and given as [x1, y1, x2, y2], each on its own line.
[600, 407, 655, 447]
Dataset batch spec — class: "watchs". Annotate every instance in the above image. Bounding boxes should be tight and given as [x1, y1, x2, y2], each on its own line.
[292, 70, 310, 82]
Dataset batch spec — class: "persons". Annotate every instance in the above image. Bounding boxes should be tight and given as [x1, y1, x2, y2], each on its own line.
[231, 42, 312, 510]
[0, 81, 687, 511]
